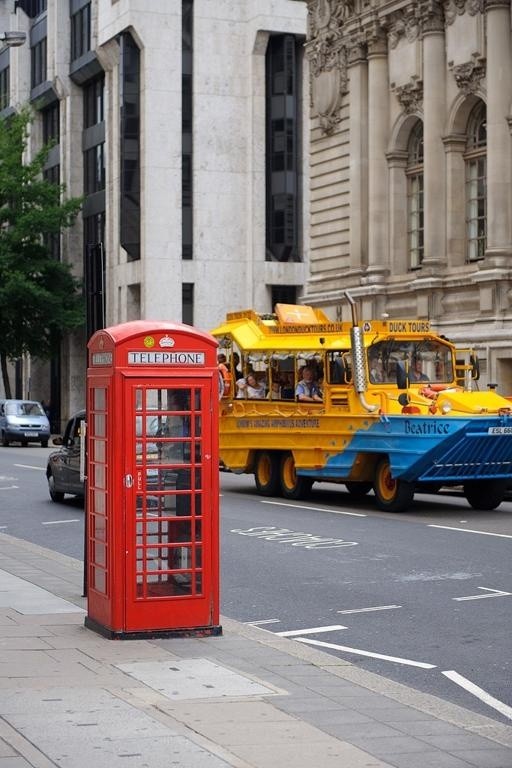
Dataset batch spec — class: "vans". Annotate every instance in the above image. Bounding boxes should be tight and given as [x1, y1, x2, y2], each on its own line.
[0, 398, 52, 449]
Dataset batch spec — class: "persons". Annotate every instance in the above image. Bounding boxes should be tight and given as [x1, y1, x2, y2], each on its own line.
[217, 345, 430, 405]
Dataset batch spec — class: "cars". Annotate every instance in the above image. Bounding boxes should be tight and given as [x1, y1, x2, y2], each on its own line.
[47, 410, 161, 507]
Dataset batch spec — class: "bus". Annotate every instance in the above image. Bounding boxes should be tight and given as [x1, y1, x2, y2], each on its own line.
[203, 288, 511, 513]
